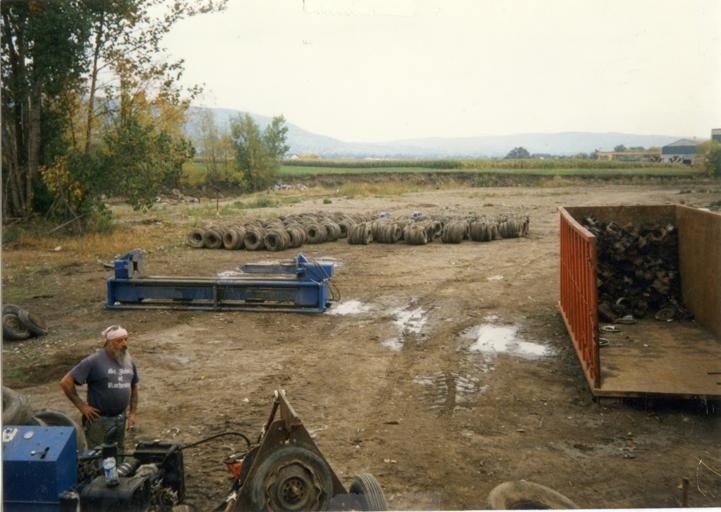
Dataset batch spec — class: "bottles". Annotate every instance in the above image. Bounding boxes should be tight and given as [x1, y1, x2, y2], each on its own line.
[102, 456, 119, 484]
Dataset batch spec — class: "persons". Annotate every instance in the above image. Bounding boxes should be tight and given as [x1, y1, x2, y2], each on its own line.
[60, 325, 139, 465]
